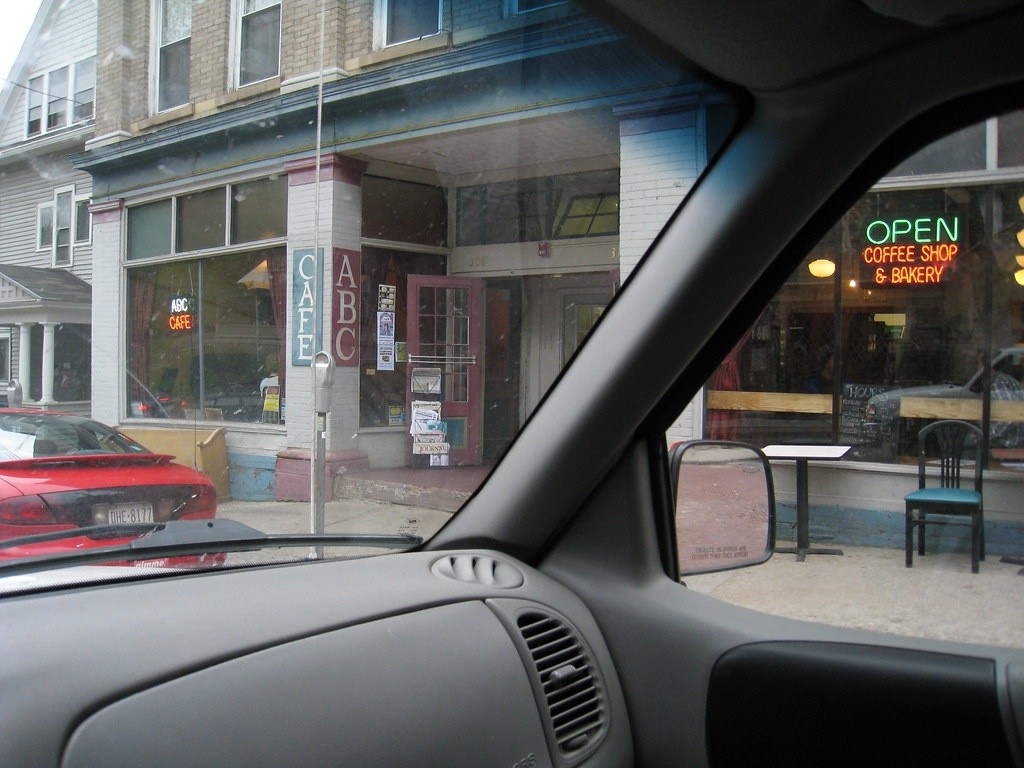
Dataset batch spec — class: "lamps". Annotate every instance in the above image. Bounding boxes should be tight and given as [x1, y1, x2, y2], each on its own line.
[809, 259, 835, 279]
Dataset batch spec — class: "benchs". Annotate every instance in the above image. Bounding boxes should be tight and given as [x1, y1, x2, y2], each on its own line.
[97, 424, 233, 505]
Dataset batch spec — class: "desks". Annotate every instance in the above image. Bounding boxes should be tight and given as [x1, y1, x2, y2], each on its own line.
[759, 445, 853, 563]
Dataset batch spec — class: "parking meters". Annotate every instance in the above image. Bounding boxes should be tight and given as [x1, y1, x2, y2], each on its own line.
[6, 379, 22, 409]
[312, 350, 337, 558]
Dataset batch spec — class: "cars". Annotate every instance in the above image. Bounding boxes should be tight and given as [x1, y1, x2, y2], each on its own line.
[0, 408, 226, 569]
[128, 384, 187, 419]
[864, 344, 1024, 458]
[204, 382, 264, 413]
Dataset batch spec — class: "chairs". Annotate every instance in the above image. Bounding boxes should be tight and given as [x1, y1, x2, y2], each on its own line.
[33, 441, 58, 457]
[903, 420, 986, 574]
